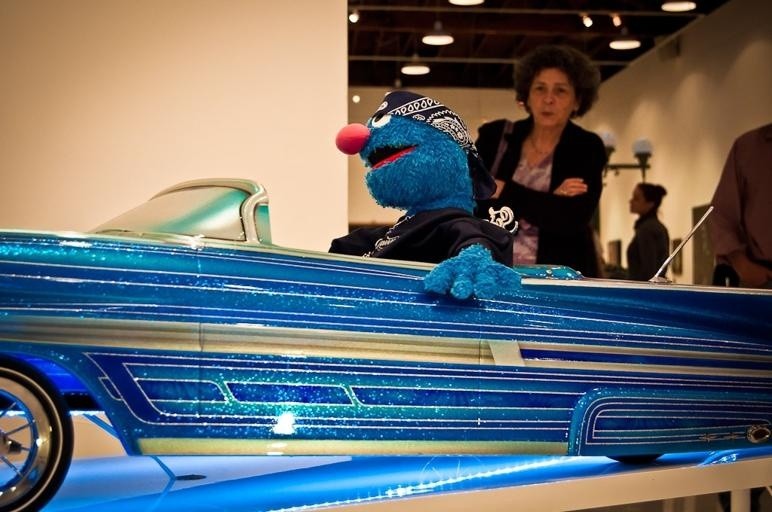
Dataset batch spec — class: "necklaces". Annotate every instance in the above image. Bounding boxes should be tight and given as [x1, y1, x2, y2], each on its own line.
[529, 134, 556, 155]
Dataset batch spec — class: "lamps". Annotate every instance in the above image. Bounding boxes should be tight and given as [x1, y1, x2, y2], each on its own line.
[609, 28, 641, 51]
[422, 21, 454, 46]
[400, 54, 431, 75]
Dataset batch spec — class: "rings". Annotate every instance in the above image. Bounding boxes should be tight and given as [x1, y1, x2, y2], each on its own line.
[560, 189, 568, 195]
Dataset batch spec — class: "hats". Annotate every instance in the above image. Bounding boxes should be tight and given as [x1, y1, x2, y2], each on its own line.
[372, 89, 497, 201]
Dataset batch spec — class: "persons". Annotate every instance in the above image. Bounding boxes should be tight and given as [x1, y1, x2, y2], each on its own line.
[705, 122, 772, 286]
[622, 181, 670, 277]
[474, 46, 605, 277]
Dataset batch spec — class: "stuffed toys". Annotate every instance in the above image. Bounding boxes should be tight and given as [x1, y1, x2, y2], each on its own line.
[337, 87, 525, 302]
